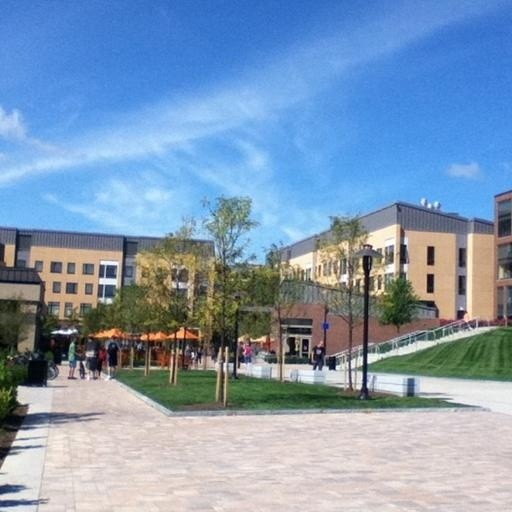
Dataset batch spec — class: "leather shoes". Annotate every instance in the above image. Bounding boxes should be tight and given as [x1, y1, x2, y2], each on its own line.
[68, 377, 77, 380]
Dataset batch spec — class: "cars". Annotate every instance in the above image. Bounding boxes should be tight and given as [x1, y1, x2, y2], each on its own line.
[256, 349, 276, 363]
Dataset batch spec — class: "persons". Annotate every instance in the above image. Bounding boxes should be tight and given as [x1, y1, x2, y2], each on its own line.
[68, 338, 119, 379]
[312, 341, 326, 370]
[238, 340, 252, 364]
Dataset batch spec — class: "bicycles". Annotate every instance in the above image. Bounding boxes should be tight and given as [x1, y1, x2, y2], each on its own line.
[76, 356, 88, 380]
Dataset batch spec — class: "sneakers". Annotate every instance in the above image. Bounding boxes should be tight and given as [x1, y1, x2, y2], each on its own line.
[87, 376, 96, 379]
[107, 376, 115, 379]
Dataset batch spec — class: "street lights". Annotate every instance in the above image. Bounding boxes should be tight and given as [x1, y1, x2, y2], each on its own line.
[182, 310, 189, 368]
[233, 289, 247, 377]
[353, 242, 384, 401]
